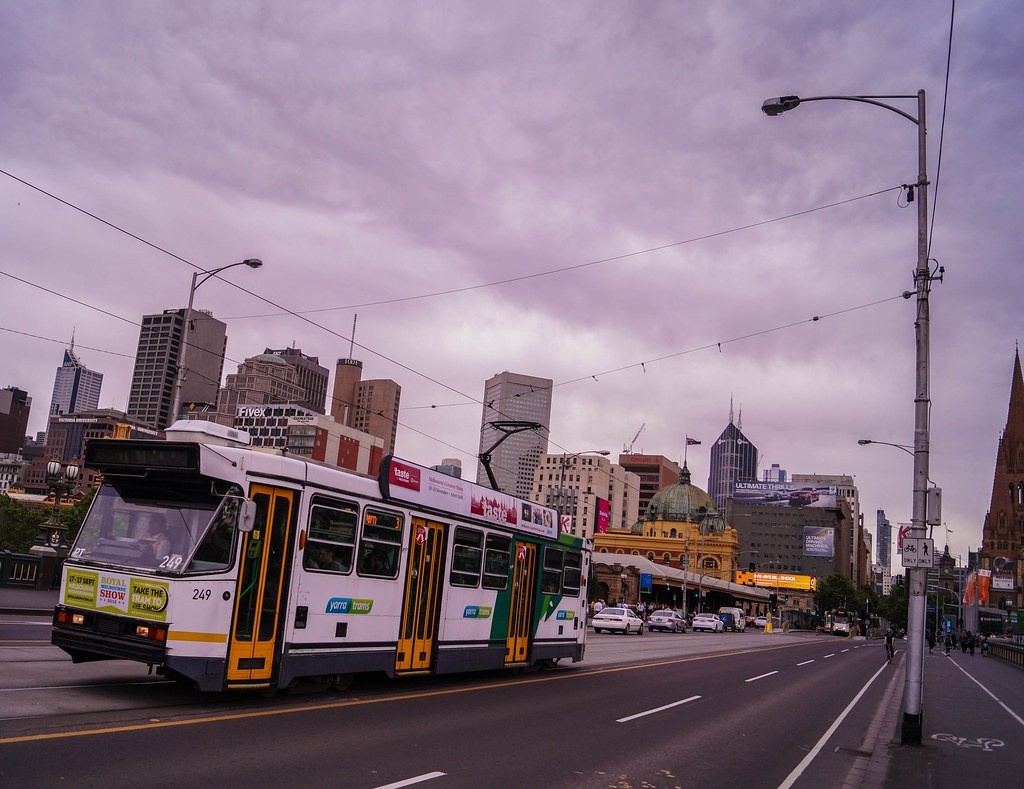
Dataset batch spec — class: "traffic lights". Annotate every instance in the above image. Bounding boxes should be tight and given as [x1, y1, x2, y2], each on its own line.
[816, 611, 819, 618]
[768, 594, 774, 604]
[749, 562, 755, 572]
[785, 595, 788, 601]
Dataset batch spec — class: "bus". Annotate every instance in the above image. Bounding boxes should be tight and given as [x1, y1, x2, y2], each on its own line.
[831, 610, 854, 636]
[50, 419, 593, 700]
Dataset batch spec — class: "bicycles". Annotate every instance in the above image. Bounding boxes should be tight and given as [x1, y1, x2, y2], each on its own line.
[936, 636, 942, 646]
[885, 643, 895, 664]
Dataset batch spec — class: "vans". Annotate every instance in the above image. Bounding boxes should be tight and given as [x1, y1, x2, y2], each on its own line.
[719, 607, 746, 632]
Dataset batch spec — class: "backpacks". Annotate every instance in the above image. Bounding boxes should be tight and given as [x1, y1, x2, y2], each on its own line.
[945, 637, 951, 647]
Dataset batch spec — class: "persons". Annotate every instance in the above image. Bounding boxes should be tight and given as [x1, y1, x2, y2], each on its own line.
[745, 617, 754, 628]
[704, 608, 720, 615]
[811, 617, 821, 630]
[608, 598, 617, 608]
[857, 620, 879, 636]
[781, 614, 784, 628]
[637, 601, 683, 621]
[936, 625, 943, 646]
[544, 576, 557, 593]
[794, 619, 801, 629]
[788, 616, 792, 629]
[617, 596, 628, 608]
[306, 545, 338, 571]
[366, 555, 389, 576]
[928, 632, 935, 654]
[776, 613, 779, 628]
[590, 598, 606, 618]
[883, 624, 896, 660]
[944, 630, 1024, 657]
[688, 607, 698, 625]
[142, 513, 171, 566]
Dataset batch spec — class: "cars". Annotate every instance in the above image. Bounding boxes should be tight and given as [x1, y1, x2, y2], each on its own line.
[648, 610, 687, 633]
[764, 489, 792, 500]
[592, 607, 644, 635]
[754, 617, 767, 629]
[788, 487, 819, 505]
[692, 613, 724, 633]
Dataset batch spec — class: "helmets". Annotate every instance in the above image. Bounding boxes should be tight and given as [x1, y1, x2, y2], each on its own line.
[887, 629, 892, 635]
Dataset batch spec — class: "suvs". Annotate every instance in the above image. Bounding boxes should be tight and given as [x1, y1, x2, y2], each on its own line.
[716, 613, 735, 631]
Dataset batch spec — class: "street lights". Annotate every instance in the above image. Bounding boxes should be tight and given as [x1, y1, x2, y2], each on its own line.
[170, 258, 263, 428]
[33, 449, 80, 556]
[682, 512, 718, 618]
[761, 90, 945, 748]
[1005, 598, 1014, 634]
[928, 529, 962, 633]
[698, 513, 751, 615]
[727, 550, 758, 608]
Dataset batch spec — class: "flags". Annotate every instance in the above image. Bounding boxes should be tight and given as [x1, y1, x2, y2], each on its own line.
[687, 437, 701, 445]
